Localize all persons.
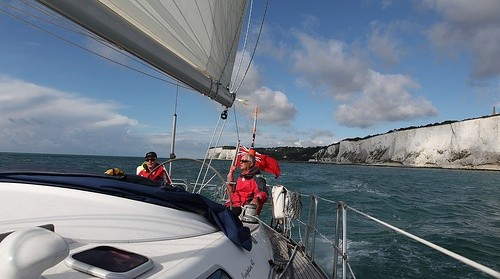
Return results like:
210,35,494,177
136,152,172,185
225,154,268,217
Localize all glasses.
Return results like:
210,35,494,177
240,160,251,163
146,158,155,162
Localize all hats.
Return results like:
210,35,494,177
144,151,157,160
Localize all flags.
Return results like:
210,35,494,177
234,144,280,179
